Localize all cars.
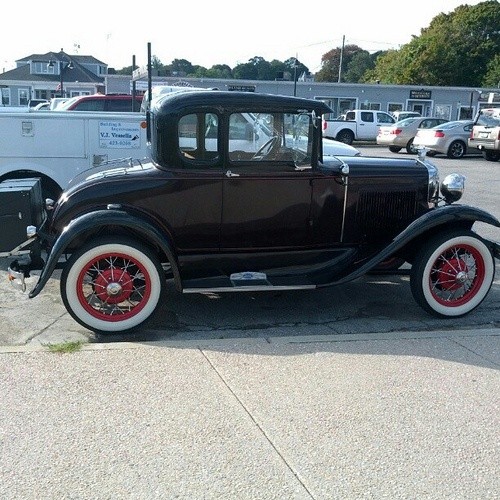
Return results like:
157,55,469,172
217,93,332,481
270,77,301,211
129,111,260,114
413,120,483,159
376,117,451,154
28,93,144,112
394,111,422,121
0,85,362,221
6,89,500,336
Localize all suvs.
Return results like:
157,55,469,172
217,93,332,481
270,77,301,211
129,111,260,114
468,108,500,160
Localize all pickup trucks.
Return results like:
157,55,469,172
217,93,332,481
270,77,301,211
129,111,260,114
322,110,398,145
